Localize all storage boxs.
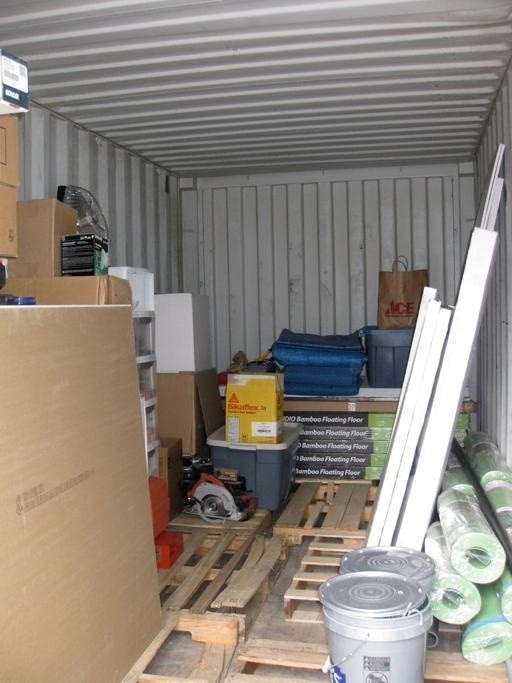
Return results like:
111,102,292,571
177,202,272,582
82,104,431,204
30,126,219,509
0,48,32,114
2,277,131,303
366,324,415,387
225,371,284,443
0,115,19,176
0,183,20,258
152,373,221,454
153,292,212,372
7,199,78,278
1,305,166,683
207,417,303,511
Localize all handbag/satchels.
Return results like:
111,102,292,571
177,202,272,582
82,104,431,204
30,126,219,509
378,255,428,329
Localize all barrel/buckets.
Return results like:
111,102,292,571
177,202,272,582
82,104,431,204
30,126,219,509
338,545,438,598
317,569,434,683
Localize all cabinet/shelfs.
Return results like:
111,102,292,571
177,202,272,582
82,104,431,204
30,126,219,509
130,310,160,478
